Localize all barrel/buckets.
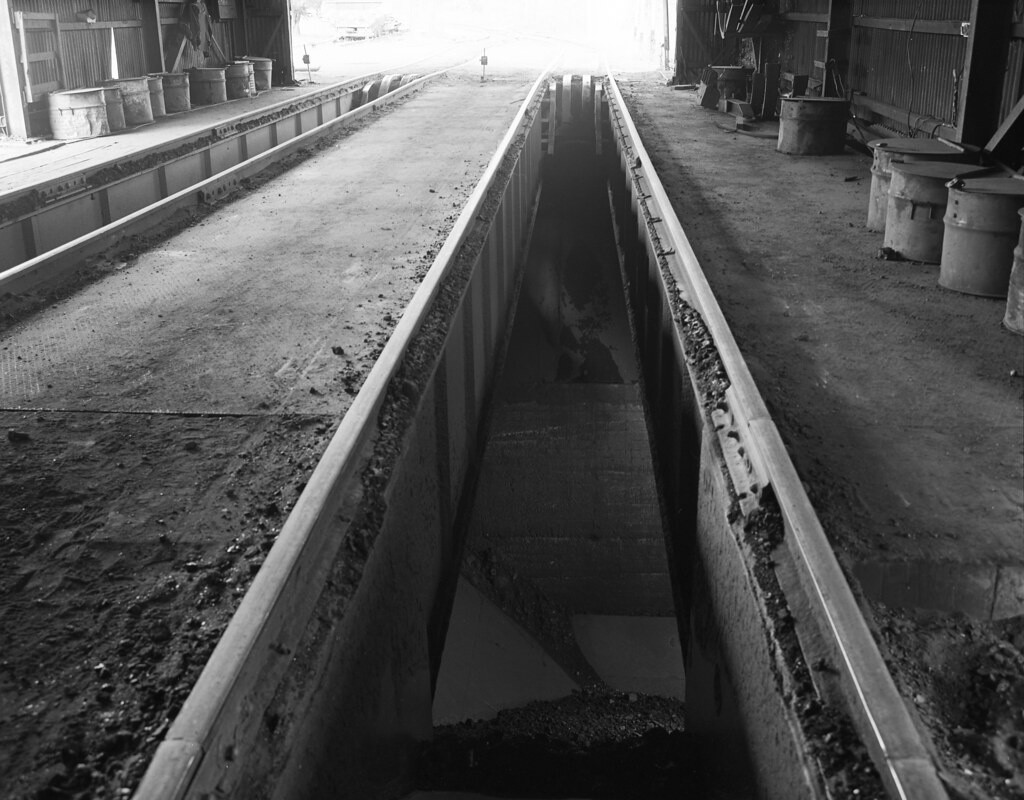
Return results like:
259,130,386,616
1002,208,1024,335
243,57,272,89
183,64,231,104
96,77,153,123
103,87,127,131
148,72,190,112
776,98,850,155
47,88,110,138
237,62,256,93
147,77,168,116
867,138,980,234
937,177,1024,296
227,65,251,98
881,159,986,264
